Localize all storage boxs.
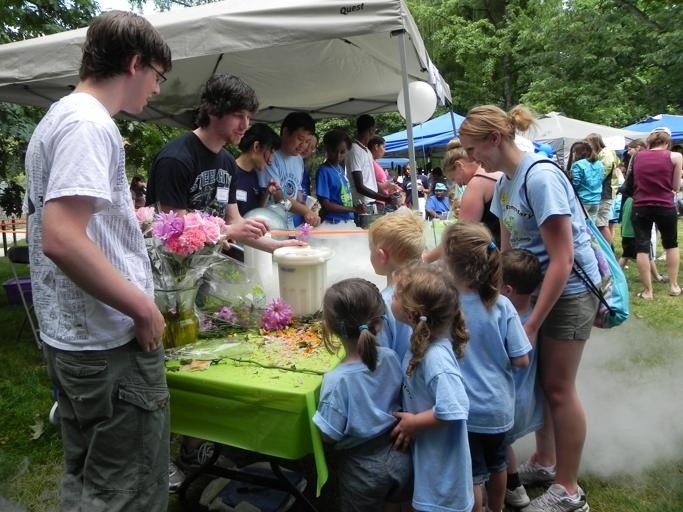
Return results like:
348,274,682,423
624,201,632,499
1,275,33,306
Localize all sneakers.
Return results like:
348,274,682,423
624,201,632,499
505,485,530,507
519,484,590,512
516,453,557,484
168,459,186,494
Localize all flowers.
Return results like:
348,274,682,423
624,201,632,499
297,222,315,244
194,290,294,340
130,202,236,348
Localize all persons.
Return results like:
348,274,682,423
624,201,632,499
129,75,321,254
313,104,629,511
24,10,172,512
567,127,682,301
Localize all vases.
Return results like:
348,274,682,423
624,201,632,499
150,279,202,353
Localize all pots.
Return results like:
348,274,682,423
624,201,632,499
359,214,382,228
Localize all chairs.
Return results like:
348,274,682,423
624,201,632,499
4,243,46,351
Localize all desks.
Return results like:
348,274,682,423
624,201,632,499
154,312,351,511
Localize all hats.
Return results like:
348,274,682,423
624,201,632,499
434,182,447,191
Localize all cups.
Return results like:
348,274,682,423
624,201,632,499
304,195,316,208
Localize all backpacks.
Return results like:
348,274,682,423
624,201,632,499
523,159,627,330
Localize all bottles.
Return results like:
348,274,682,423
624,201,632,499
391,191,400,210
417,196,426,220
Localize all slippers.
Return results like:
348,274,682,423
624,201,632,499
637,274,681,300
